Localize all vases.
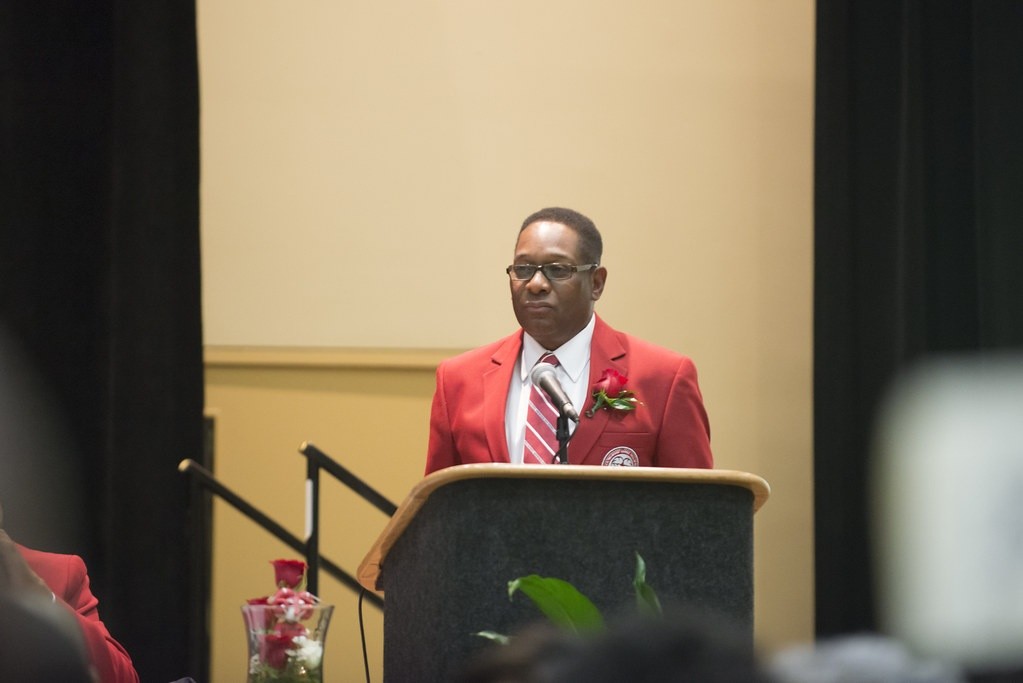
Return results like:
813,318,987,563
239,601,334,683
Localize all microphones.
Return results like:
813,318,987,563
531,363,580,423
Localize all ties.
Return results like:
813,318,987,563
523,354,561,463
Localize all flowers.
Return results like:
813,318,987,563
586,366,643,418
239,556,331,683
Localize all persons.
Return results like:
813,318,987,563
423,208,713,476
0,531,138,683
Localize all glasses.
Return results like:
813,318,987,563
506,262,598,281
